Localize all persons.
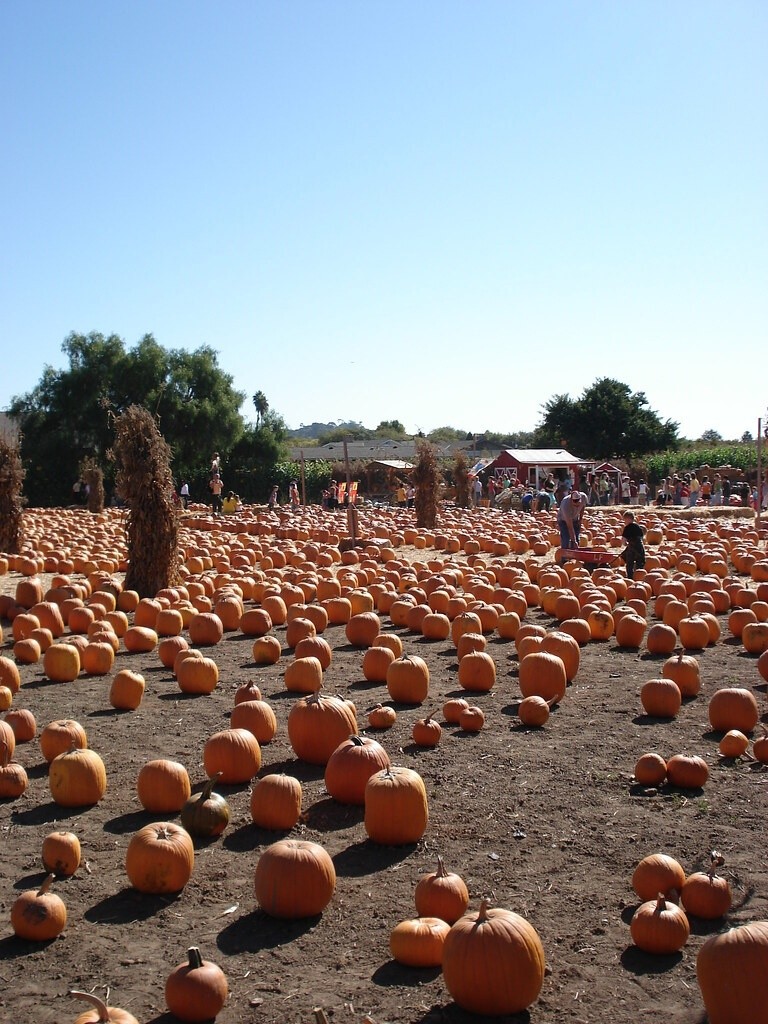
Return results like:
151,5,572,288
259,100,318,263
578,472,650,506
536,492,550,513
269,485,279,511
540,472,573,509
180,480,189,507
557,490,588,561
622,510,645,579
397,484,406,508
73,481,90,503
522,491,533,513
322,480,339,511
408,485,416,508
657,473,768,512
289,478,300,511
474,472,521,501
223,491,242,513
209,474,224,512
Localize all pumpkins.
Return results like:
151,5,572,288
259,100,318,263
697,920,768,1024
0,503,768,966
166,946,228,1018
69,989,138,1024
441,896,544,1012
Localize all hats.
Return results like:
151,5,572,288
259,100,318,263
571,491,581,499
